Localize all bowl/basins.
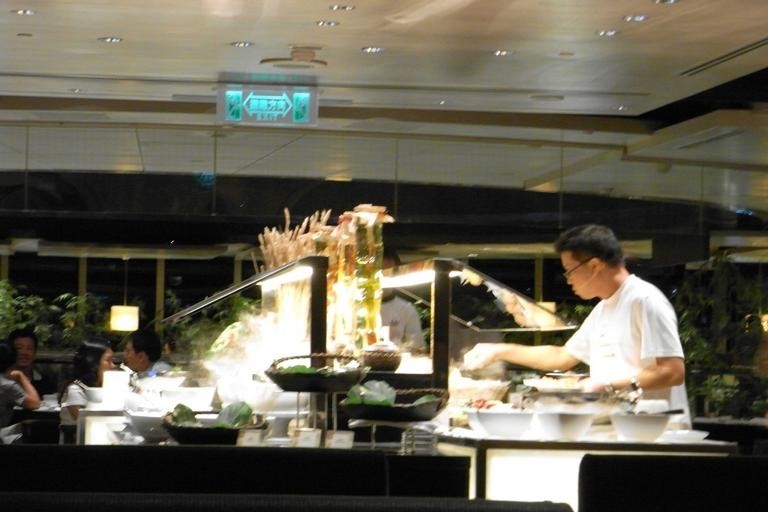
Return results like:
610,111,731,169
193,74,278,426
84,372,445,453
38,392,64,411
451,365,710,441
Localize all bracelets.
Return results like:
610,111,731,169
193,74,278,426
606,380,612,392
630,378,641,392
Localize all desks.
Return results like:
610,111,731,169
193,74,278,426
75,404,221,445
434,432,740,501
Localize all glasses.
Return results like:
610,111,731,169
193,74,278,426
562,255,599,281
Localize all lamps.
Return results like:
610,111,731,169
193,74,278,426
108,259,142,333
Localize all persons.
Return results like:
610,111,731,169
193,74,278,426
459,223,692,431
0,329,173,445
380,247,424,351
